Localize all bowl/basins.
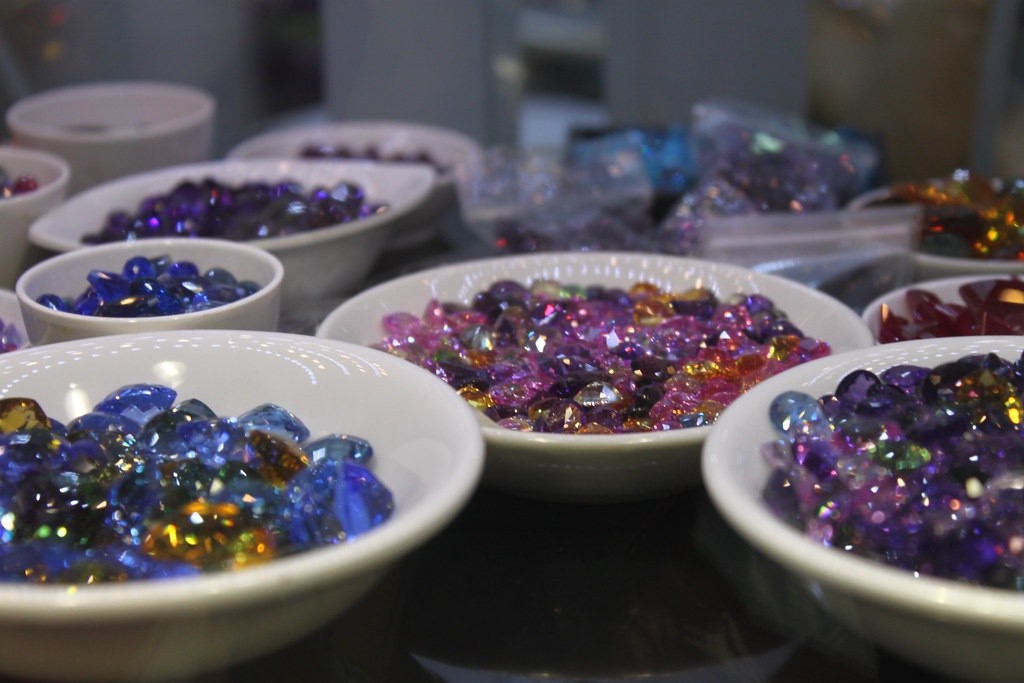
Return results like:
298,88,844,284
0,83,1024,683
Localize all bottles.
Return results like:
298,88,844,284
498,11,613,157
